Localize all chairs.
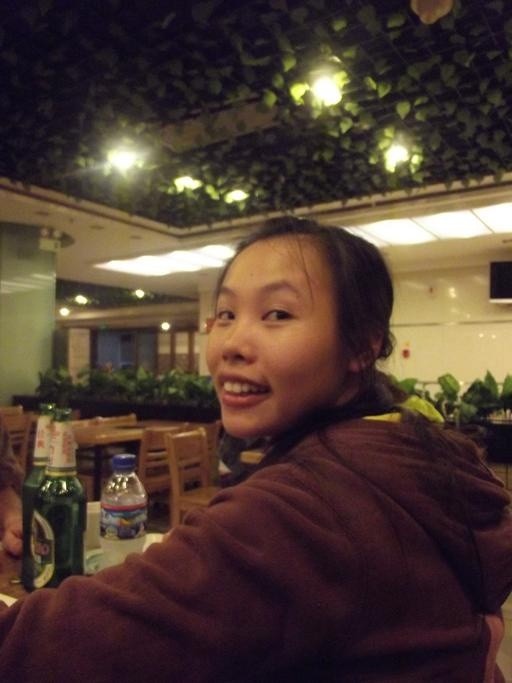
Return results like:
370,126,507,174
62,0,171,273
1,401,223,531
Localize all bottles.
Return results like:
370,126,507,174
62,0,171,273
20,403,150,594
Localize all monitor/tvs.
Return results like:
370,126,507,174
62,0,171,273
488,260,512,305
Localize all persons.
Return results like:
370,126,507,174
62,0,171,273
0,211,511,682
0,411,47,578
